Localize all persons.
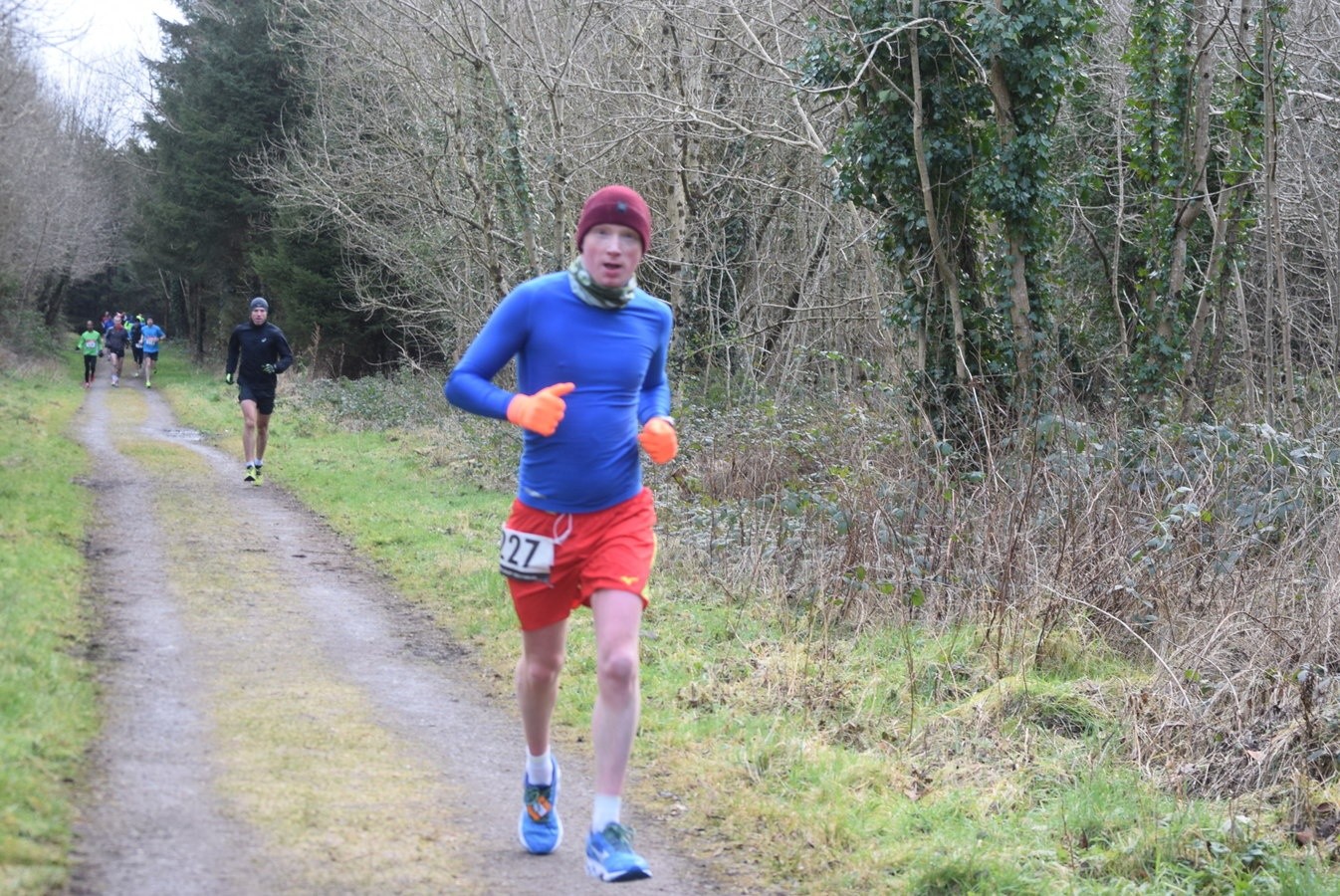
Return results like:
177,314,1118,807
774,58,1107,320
99,308,149,377
74,318,104,388
224,296,292,482
439,183,680,881
102,314,131,387
136,317,167,389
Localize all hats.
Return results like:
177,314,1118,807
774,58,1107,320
250,297,269,312
577,185,652,254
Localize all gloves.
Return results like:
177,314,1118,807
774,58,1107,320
262,363,276,375
639,417,678,466
75,347,79,351
98,350,103,357
226,373,234,384
505,383,575,437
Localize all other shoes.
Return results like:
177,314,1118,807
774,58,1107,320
111,381,119,387
135,372,140,377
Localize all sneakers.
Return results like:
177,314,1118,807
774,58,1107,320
90,375,94,382
244,465,256,482
146,382,151,388
518,753,563,855
585,822,652,882
254,466,264,486
85,382,90,389
153,369,158,374
